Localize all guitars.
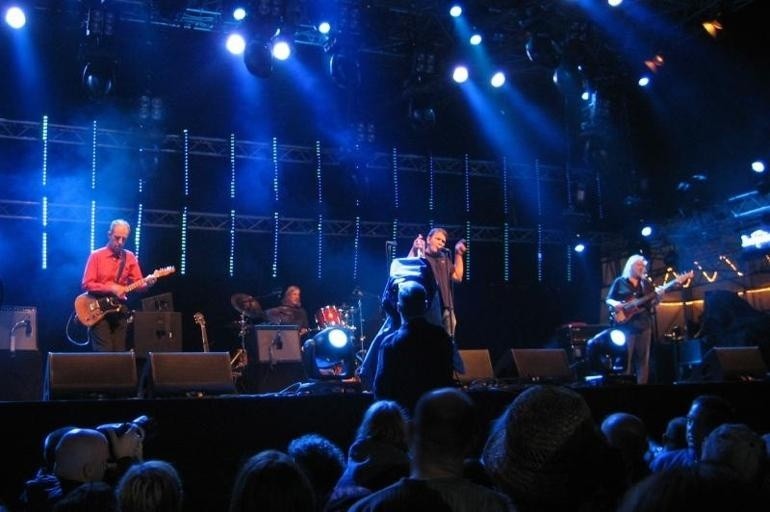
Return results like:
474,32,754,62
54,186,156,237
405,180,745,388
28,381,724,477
74,266,175,326
608,271,696,323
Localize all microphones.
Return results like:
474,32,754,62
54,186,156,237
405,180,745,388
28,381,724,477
437,246,449,251
644,272,656,287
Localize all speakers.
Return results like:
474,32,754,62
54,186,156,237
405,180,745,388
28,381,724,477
704,347,766,380
255,325,303,364
140,352,234,394
0,305,38,352
453,349,497,382
44,352,137,400
509,348,572,380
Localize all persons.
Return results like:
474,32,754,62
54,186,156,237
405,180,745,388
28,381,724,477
372,279,466,417
605,253,666,387
406,226,468,373
261,284,310,344
19,382,769,511
79,218,158,351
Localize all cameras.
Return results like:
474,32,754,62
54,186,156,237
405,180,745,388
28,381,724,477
96,414,151,442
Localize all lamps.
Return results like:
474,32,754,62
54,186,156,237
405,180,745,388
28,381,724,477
563,229,588,254
750,149,768,188
301,323,355,382
635,212,659,243
441,3,508,90
220,1,292,81
584,329,630,385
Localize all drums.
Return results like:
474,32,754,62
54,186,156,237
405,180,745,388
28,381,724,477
315,305,345,328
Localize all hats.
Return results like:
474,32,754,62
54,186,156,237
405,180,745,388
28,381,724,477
485,383,618,503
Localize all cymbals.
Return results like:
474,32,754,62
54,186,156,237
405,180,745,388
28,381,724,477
232,294,262,317
